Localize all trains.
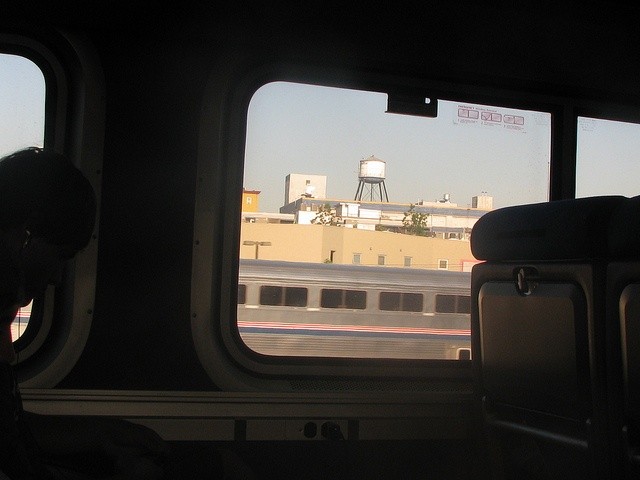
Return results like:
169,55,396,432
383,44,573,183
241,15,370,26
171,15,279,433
0,0,640,479
237,258,472,361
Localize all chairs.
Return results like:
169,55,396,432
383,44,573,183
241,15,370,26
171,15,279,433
618,195,639,478
470,195,632,479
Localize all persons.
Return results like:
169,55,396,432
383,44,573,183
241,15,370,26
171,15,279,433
1,145,172,480
